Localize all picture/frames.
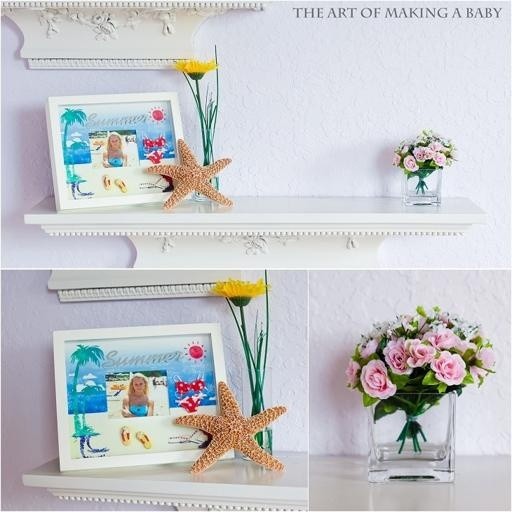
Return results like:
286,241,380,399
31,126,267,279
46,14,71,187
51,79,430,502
42,93,193,212
54,323,234,472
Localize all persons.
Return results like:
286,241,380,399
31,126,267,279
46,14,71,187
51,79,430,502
120,373,155,417
100,132,128,168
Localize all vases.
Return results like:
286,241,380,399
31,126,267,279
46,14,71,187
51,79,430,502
194,129,224,204
401,169,443,207
366,392,457,484
240,369,274,461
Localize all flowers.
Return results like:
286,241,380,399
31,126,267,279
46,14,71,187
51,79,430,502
172,43,226,193
206,270,273,456
392,128,459,196
342,306,498,458
